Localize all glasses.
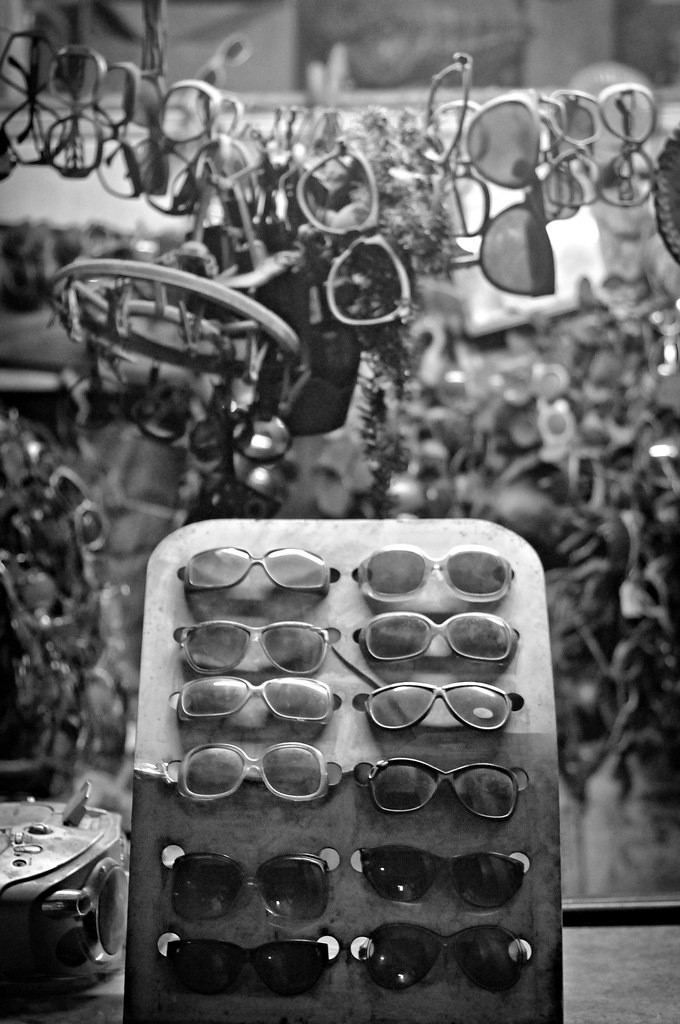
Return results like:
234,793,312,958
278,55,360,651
183,544,330,597
360,844,524,908
358,923,526,991
176,674,333,727
0,340,680,794
364,681,513,730
170,851,329,922
0,24,661,334
366,757,518,820
357,611,516,662
356,544,512,603
178,742,329,801
181,619,329,675
166,938,328,996
